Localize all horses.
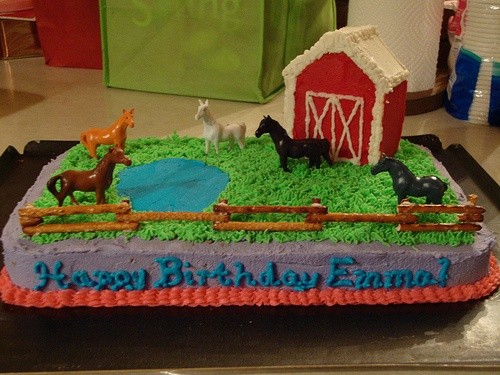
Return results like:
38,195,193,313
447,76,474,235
46,146,132,207
194,98,247,154
371,155,448,205
255,115,335,171
80,107,136,159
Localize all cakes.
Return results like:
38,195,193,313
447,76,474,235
0,27,496,292
